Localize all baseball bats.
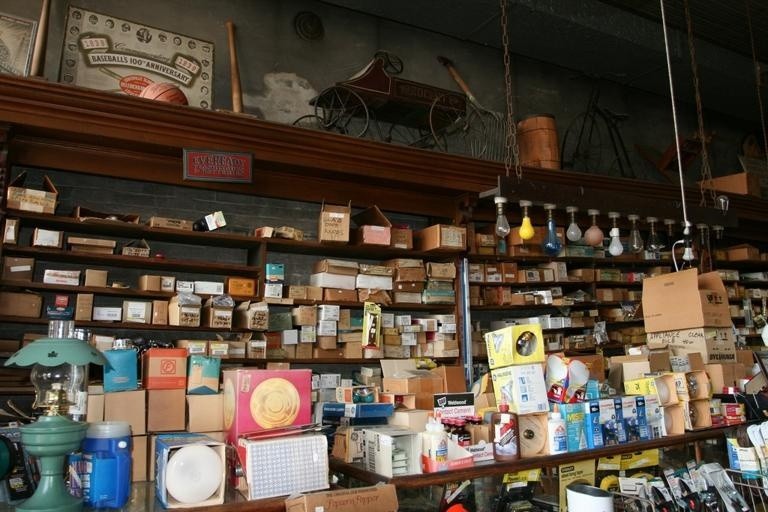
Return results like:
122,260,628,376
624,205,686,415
226,20,245,115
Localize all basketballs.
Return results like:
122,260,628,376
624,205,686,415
139,82,188,106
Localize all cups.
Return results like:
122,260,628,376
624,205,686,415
85,420,133,508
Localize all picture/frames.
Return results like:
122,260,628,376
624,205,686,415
57,3,215,112
0,12,38,79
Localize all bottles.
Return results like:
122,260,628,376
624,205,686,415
491,404,522,463
423,414,483,462
